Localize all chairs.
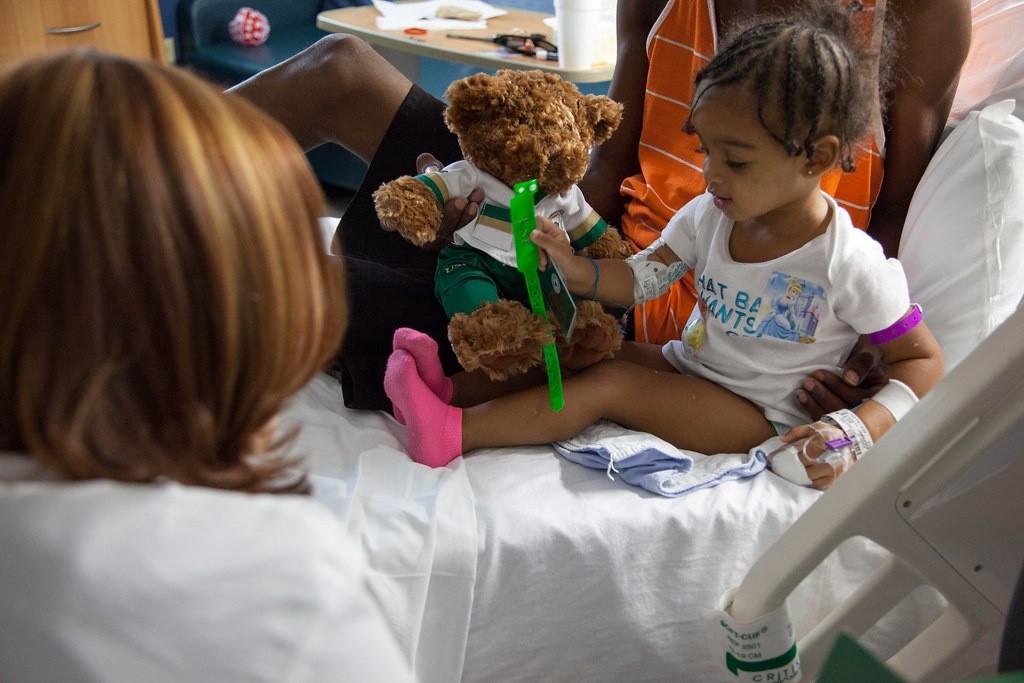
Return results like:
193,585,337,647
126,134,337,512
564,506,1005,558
179,0,336,87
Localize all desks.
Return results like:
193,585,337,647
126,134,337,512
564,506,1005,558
318,0,624,86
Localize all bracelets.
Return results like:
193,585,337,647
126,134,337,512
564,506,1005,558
823,408,873,460
574,256,599,299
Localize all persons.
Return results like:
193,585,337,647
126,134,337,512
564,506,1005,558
385,14,944,491
211,0,970,422
0,55,423,683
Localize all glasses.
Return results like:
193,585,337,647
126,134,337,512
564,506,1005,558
493,34,558,57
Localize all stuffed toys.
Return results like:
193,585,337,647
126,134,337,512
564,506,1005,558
371,71,633,382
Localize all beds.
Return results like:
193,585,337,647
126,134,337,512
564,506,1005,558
262,216,1024,683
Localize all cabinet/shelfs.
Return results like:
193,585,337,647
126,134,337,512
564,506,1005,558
0,0,168,76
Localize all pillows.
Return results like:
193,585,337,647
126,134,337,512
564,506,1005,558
886,96,1024,374
